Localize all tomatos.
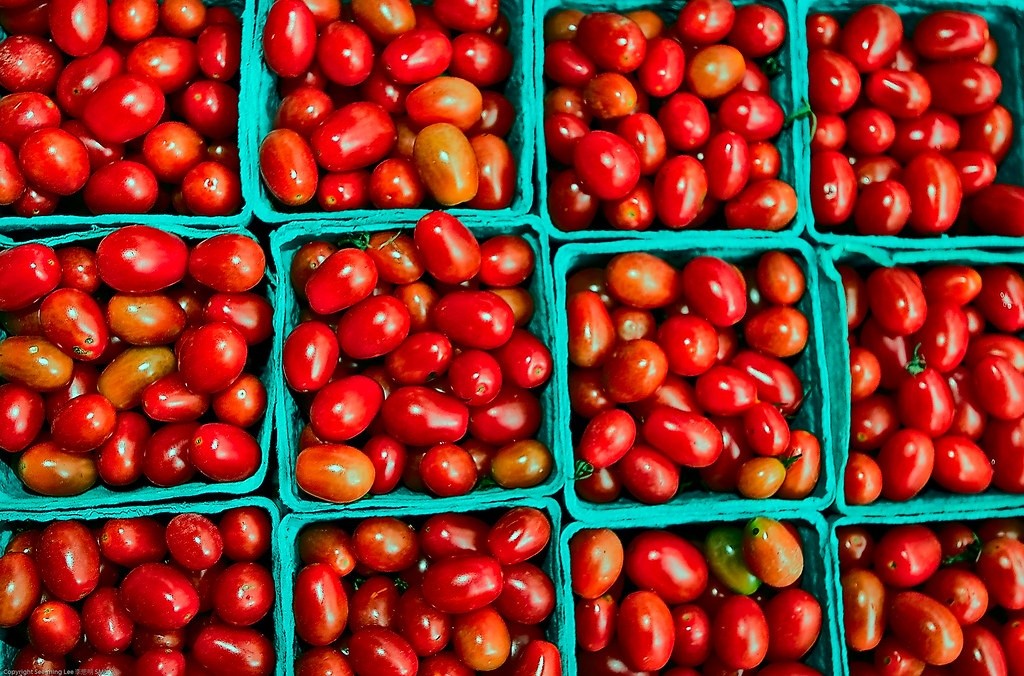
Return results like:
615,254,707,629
0,0,1024,676
